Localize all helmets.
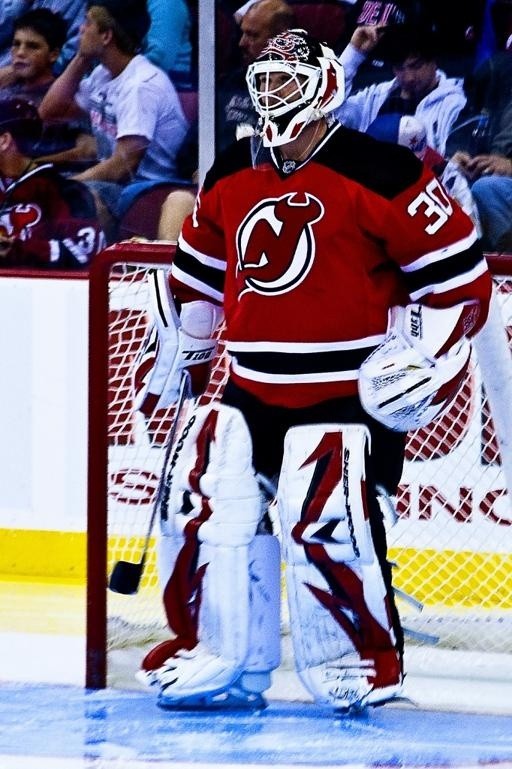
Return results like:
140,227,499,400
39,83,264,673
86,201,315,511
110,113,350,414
248,28,348,149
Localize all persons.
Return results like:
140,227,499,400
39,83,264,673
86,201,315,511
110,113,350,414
128,25,493,721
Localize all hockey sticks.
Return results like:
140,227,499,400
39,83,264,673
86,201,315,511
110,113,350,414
111,377,185,594
